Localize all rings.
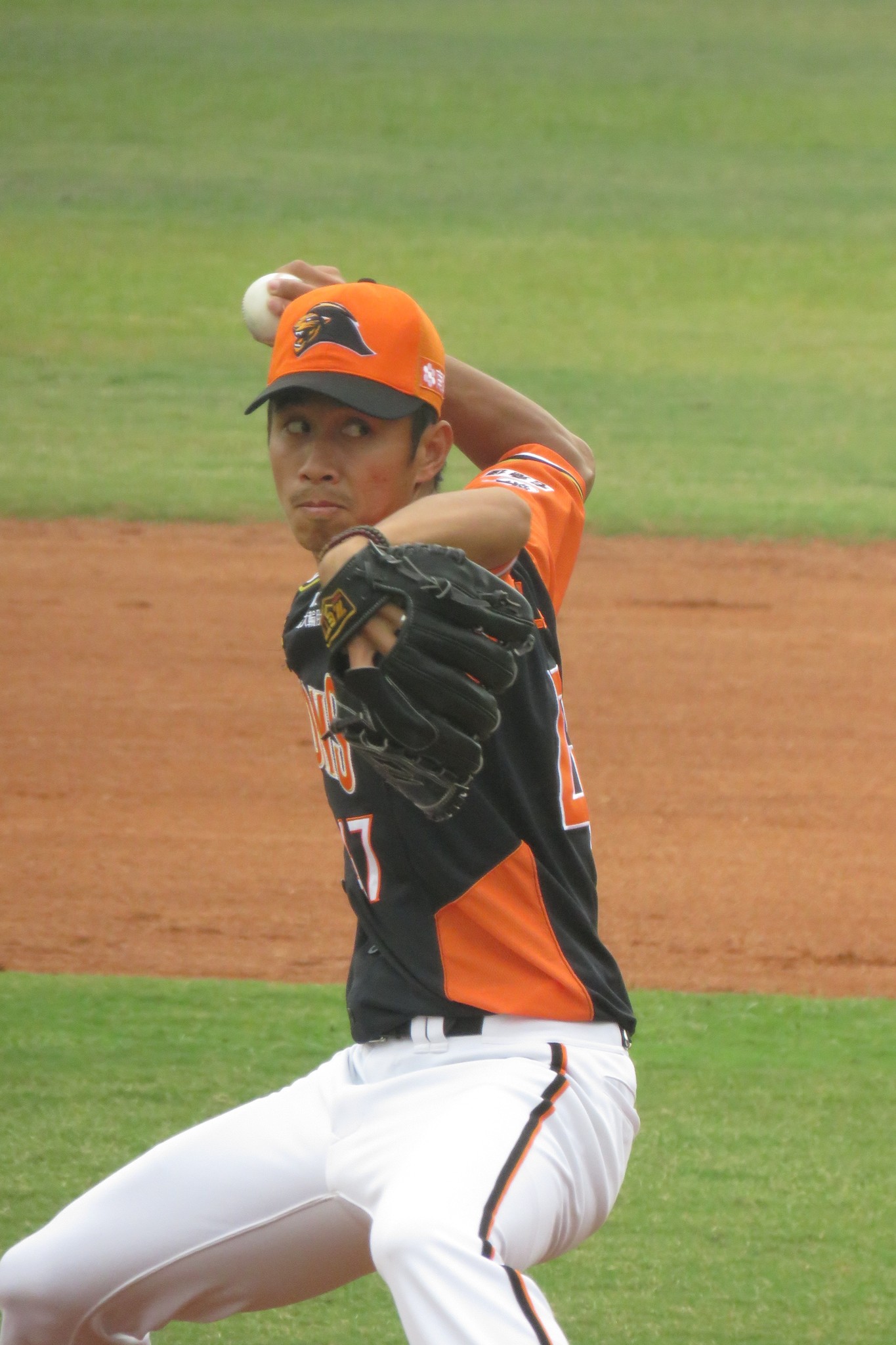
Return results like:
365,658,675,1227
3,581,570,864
397,612,407,632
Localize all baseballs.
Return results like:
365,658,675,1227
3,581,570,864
240,272,304,344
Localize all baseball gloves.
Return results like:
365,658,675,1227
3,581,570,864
315,539,534,823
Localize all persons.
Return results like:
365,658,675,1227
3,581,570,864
0,258,641,1345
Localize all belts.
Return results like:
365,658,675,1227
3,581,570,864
381,1013,631,1050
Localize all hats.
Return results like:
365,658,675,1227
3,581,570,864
243,277,447,420
